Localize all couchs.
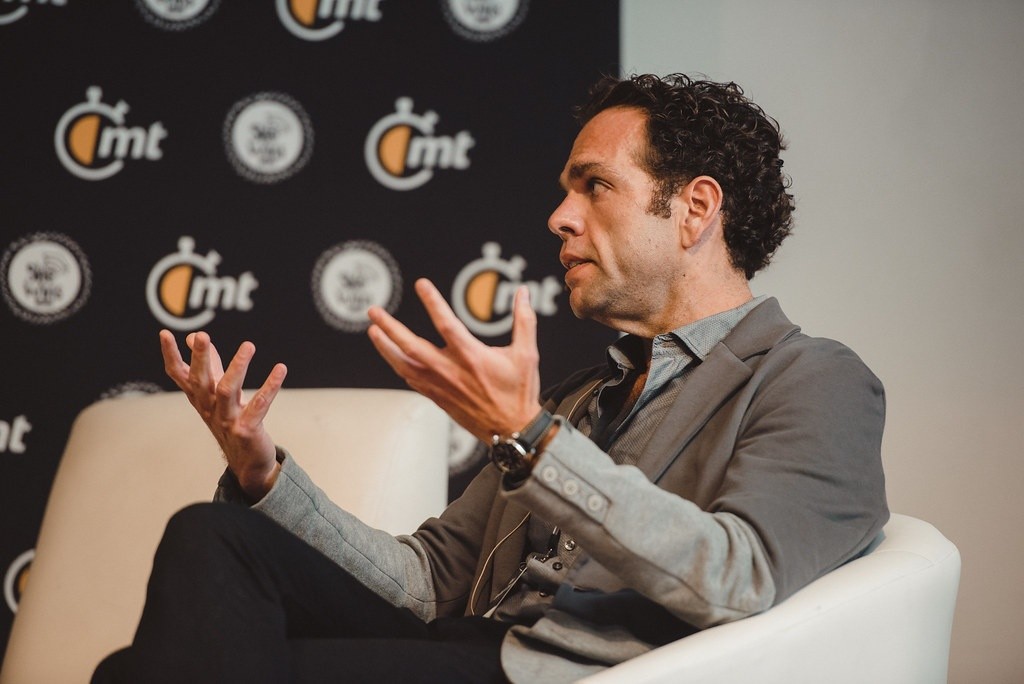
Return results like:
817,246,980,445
578,513,962,684
0,390,451,684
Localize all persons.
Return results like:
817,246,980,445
89,71,891,684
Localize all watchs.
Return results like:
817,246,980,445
487,408,554,473
